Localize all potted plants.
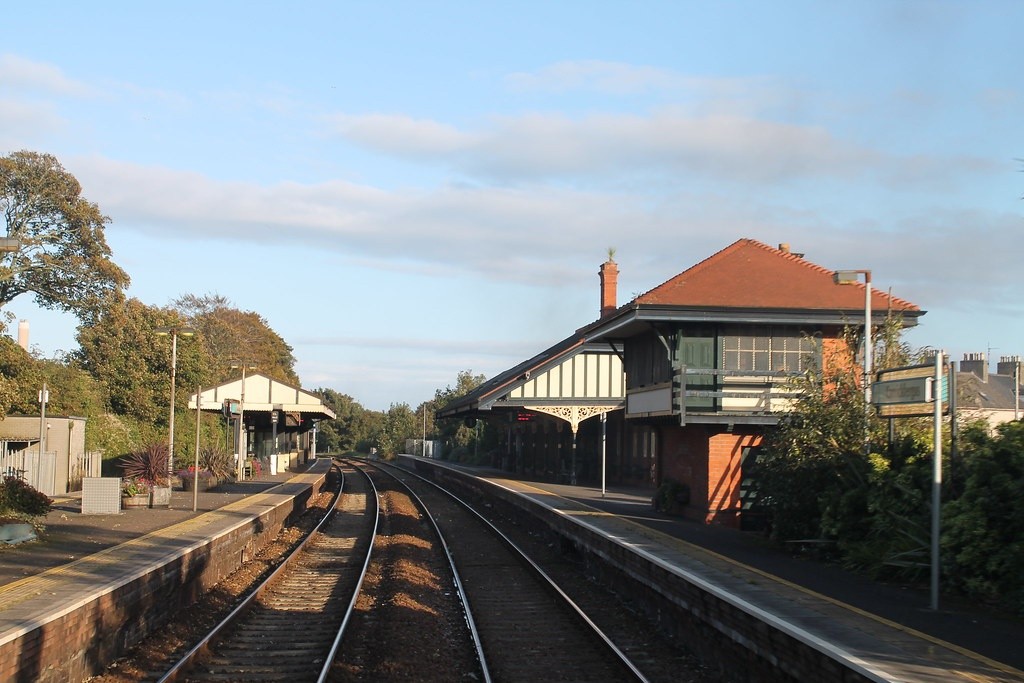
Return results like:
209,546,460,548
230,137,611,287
116,440,181,509
199,446,234,491
246,456,252,467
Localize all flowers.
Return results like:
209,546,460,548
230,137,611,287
176,466,201,480
189,470,212,479
123,478,156,496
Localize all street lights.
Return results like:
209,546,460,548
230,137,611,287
230,361,258,484
152,326,193,497
830,268,876,442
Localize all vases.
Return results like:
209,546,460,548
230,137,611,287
191,477,217,491
182,479,191,492
121,493,150,510
